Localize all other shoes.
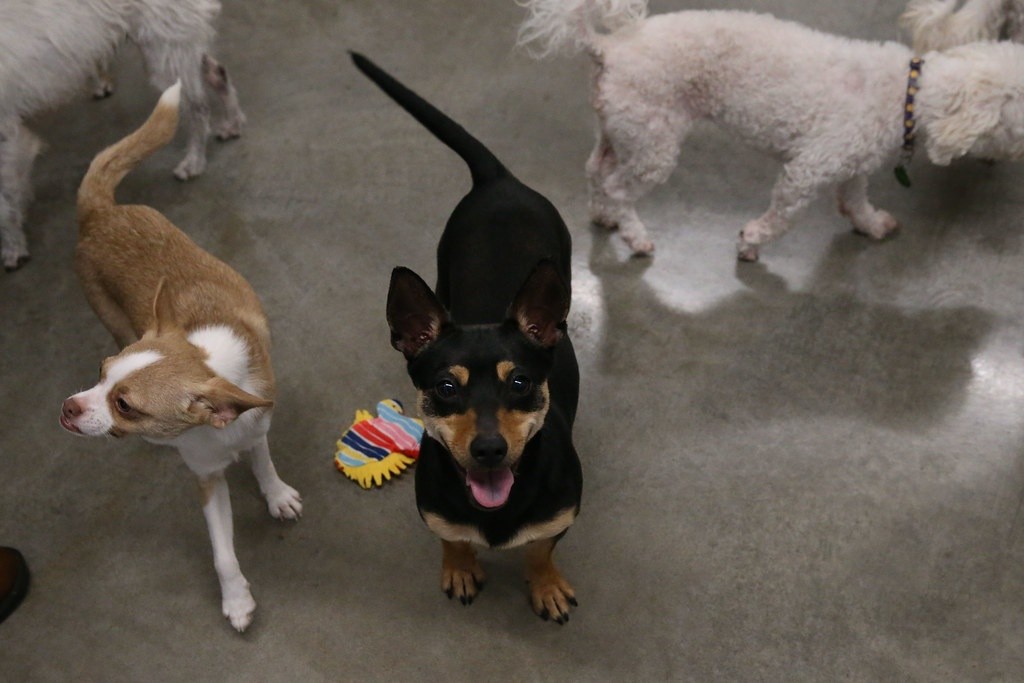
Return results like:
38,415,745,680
0,546,29,622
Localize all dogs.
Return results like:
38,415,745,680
339,47,586,628
509,1,1024,265
1,0,306,636
